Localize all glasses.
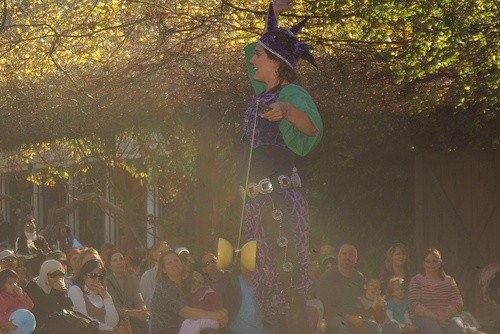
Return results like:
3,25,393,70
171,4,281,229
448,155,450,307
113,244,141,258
88,272,103,281
47,273,65,278
203,258,217,266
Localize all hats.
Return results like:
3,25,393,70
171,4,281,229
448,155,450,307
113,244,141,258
256,2,319,71
1,249,17,262
39,259,66,284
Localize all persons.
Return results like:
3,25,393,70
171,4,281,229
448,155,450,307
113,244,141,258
385,277,418,334
68,260,120,334
374,242,418,294
343,274,389,334
35,259,74,334
0,212,263,334
0,269,35,334
316,241,384,334
150,250,227,334
407,248,465,334
237,1,324,334
103,250,150,334
199,252,232,295
